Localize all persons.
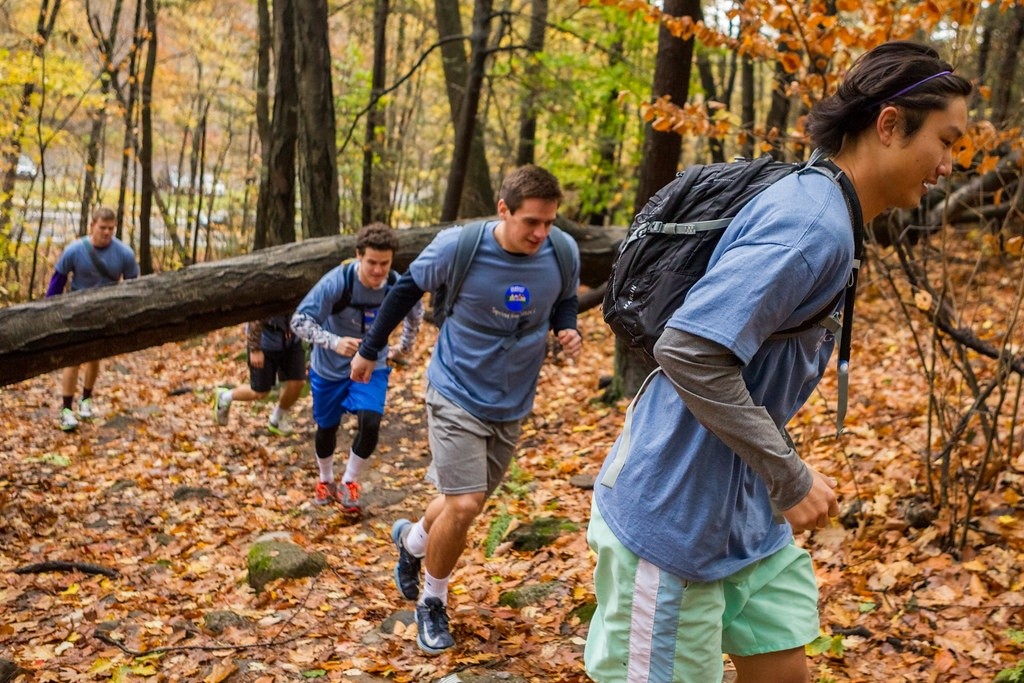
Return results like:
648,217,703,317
349,163,583,656
292,223,425,514
585,41,973,683
46,208,139,430
213,314,306,437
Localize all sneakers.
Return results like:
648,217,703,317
315,479,337,506
58,408,79,431
414,596,458,655
267,416,297,438
213,387,231,426
81,397,94,419
337,477,362,513
391,518,423,601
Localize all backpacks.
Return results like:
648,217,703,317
596,154,854,439
428,219,571,335
